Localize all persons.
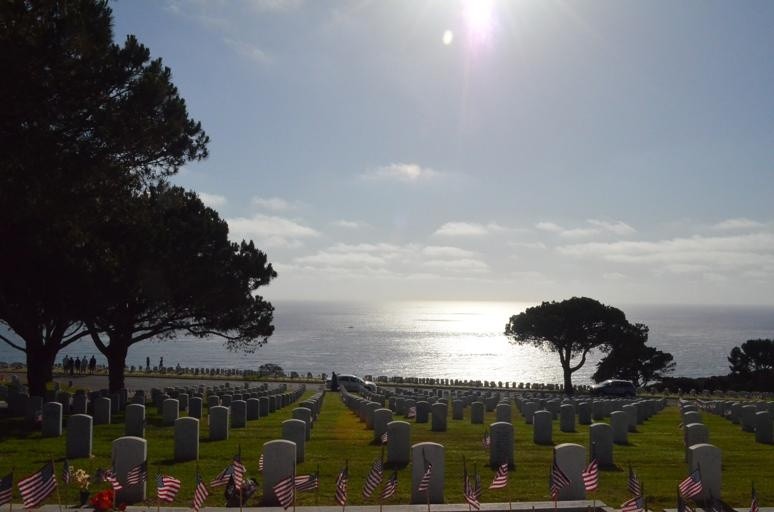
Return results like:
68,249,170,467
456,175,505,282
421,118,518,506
62,354,68,374
81,355,87,374
328,371,338,393
68,357,73,374
75,356,80,374
89,354,95,373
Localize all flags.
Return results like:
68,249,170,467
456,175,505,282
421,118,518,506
418,460,432,491
33,409,42,422
678,468,703,499
487,463,509,489
481,426,490,446
257,452,264,472
748,485,760,512
380,430,391,443
581,458,600,491
362,456,398,499
550,462,570,499
618,470,644,511
407,406,416,417
274,469,350,509
464,472,482,509
1,455,257,511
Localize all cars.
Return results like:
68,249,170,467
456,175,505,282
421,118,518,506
325,374,377,394
587,378,636,398
